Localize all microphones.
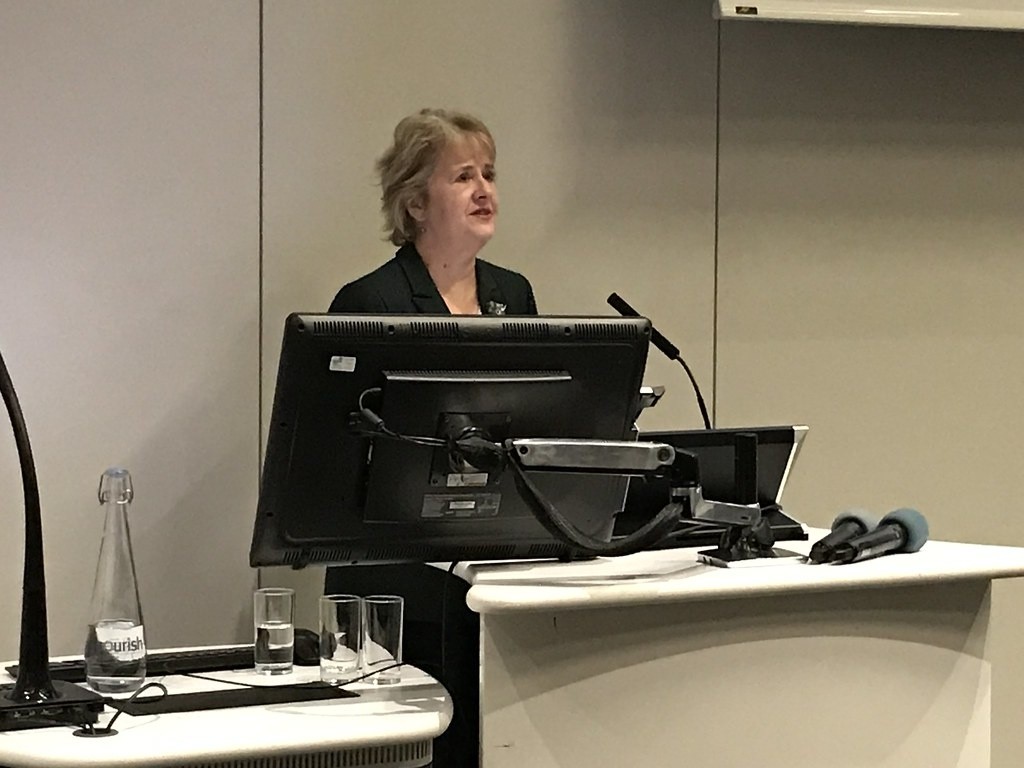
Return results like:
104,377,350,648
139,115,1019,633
810,510,875,562
607,292,711,431
834,507,929,563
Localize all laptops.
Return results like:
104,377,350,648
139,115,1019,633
610,425,811,547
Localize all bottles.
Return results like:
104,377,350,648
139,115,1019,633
85,470,147,694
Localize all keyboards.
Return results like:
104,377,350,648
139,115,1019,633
6,647,258,685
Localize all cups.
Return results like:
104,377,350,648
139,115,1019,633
318,595,361,687
361,596,405,685
253,587,296,675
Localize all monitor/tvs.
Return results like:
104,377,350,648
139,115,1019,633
250,312,653,570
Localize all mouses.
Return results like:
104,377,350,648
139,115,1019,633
293,628,320,666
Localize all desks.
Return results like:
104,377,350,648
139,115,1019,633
426,532,1024,767
0,642,453,768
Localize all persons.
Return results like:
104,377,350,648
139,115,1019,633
322,106,541,768
